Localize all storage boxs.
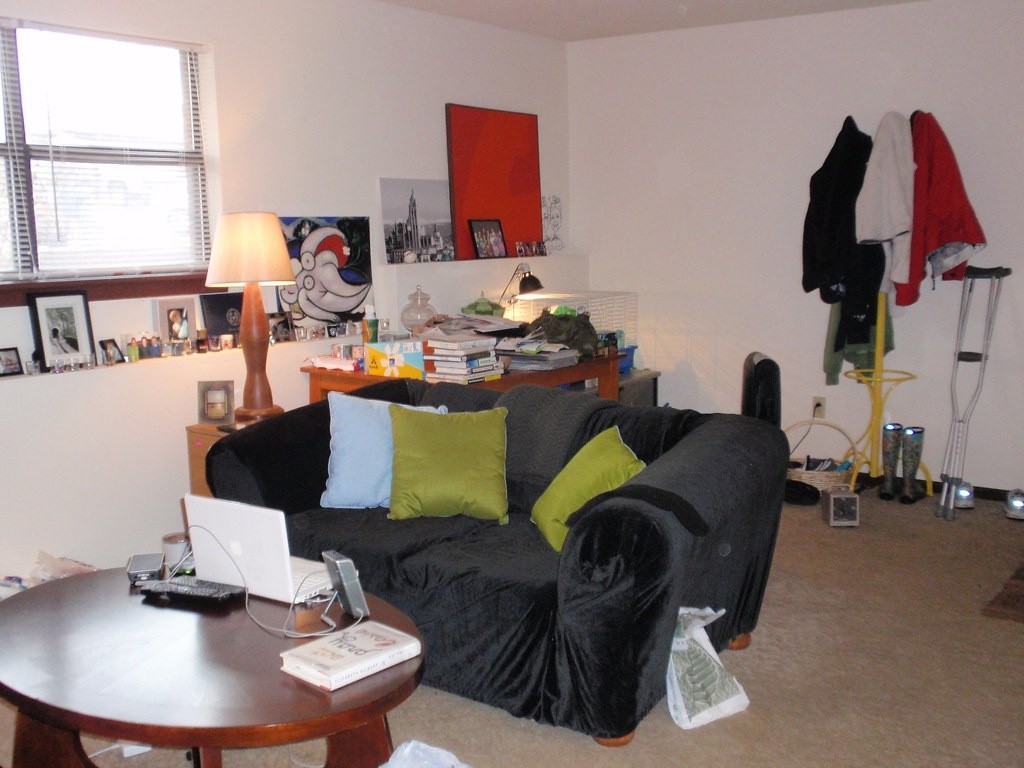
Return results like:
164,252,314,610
617,345,638,373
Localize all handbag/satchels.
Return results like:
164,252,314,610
665,607,749,729
529,305,598,356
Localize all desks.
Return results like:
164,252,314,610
0,567,425,768
618,370,661,406
301,351,625,403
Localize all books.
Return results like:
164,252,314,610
421,333,506,385
280,620,422,691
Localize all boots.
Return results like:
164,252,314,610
899,426,925,503
880,423,901,499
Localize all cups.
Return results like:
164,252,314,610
25,360,40,375
48,359,65,374
294,328,307,341
101,349,115,365
220,334,233,351
64,355,81,373
81,353,95,370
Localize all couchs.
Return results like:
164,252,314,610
207,378,788,745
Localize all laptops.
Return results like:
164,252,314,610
184,494,359,604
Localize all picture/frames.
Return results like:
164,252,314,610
469,219,509,259
99,337,125,364
26,289,99,372
0,347,24,376
157,298,197,344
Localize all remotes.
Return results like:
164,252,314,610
139,574,247,605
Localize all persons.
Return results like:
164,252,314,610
127,336,162,361
167,310,188,341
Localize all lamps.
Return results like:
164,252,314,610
498,262,544,304
205,212,297,430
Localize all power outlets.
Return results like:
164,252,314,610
813,396,825,419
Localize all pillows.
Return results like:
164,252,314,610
320,391,447,509
386,403,511,526
531,425,648,552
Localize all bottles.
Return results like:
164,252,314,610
362,304,379,344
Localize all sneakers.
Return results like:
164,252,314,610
954,482,975,508
1004,489,1024,519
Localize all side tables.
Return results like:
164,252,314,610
186,422,235,497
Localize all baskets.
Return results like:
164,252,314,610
783,421,857,493
